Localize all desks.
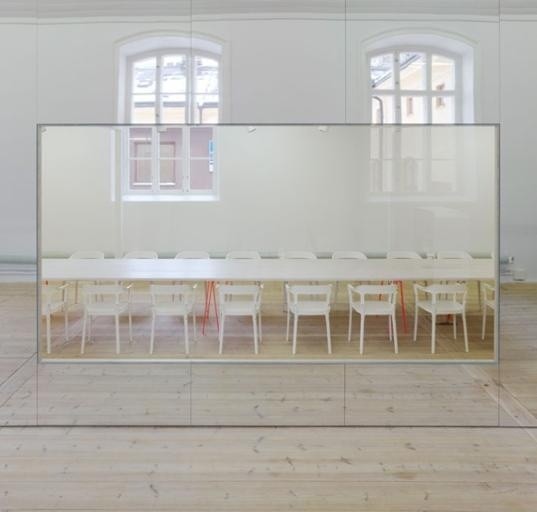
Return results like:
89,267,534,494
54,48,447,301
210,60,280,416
39,260,493,339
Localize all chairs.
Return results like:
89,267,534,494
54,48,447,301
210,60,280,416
481,283,494,341
346,282,400,354
170,249,212,306
215,283,264,354
38,283,71,354
386,250,429,303
412,282,471,354
149,282,199,354
330,250,371,304
223,250,264,304
64,249,105,306
277,249,320,302
434,249,478,302
79,283,135,355
118,249,160,304
284,283,333,355
